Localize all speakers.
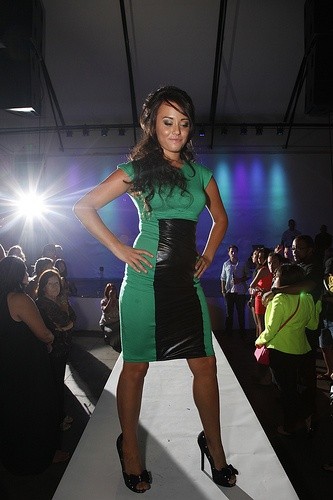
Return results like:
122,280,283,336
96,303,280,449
0,0,45,119
304,0,333,116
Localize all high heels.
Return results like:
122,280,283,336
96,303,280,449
197,430,239,488
116,433,152,493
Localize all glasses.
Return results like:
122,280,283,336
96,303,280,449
290,247,306,252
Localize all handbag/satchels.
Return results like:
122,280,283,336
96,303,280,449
254,344,270,367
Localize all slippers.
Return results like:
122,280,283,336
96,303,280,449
317,373,331,380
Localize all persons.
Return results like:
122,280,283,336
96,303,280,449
99,283,120,346
246,218,333,469
221,244,253,339
72,85,240,493
0,241,77,466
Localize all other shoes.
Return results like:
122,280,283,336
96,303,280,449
304,423,319,440
281,426,296,440
